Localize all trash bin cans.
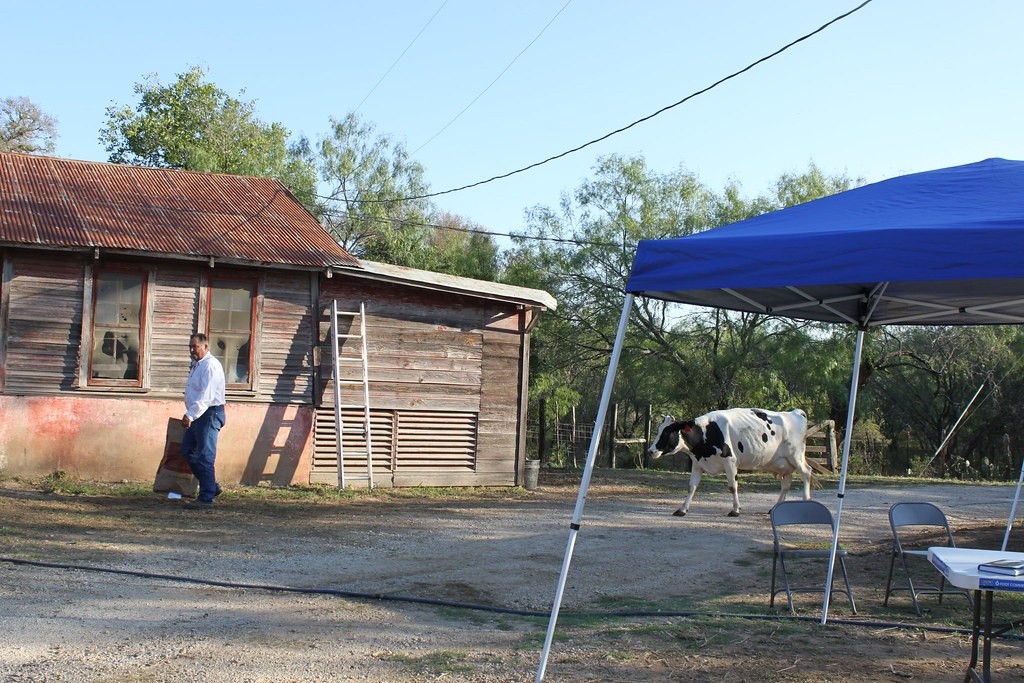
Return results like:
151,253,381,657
523,458,541,490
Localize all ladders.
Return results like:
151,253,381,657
327,297,375,490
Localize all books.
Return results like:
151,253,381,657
978,556,1024,578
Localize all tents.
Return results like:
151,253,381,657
534,155,1024,682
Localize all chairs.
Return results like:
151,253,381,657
884,500,974,618
770,500,857,617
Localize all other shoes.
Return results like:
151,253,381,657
184,500,214,509
214,483,223,496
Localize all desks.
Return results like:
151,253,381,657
925,546,1024,683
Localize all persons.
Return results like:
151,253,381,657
181,333,226,509
